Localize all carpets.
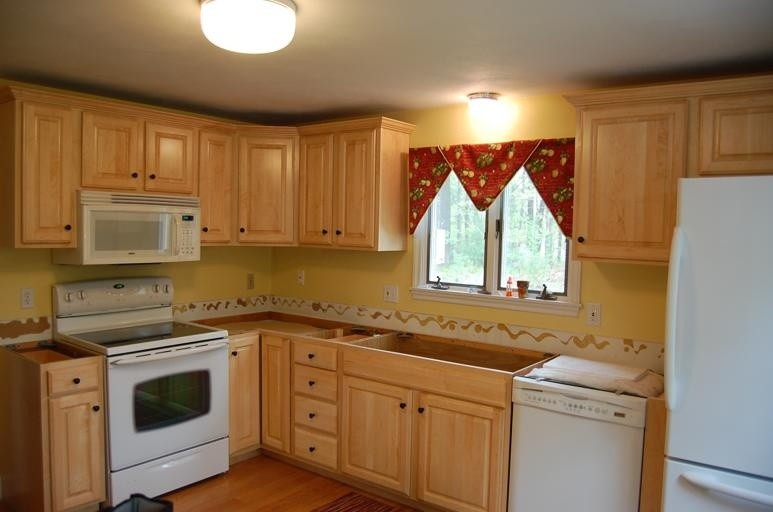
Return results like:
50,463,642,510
311,490,410,512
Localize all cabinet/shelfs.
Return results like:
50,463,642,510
695,74,773,176
75,92,198,197
561,81,694,267
1,83,79,250
298,116,416,254
235,121,297,246
293,320,394,483
343,332,561,512
194,114,235,247
1,338,109,512
191,316,327,468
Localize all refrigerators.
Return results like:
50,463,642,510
661,174,773,512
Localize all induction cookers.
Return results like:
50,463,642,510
56,305,229,359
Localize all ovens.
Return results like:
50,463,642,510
101,338,231,510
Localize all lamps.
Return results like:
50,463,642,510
468,90,506,136
198,0,299,56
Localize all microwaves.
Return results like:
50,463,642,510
50,189,203,267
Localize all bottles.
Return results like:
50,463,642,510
505,276,513,297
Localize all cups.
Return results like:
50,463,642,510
517,280,530,298
